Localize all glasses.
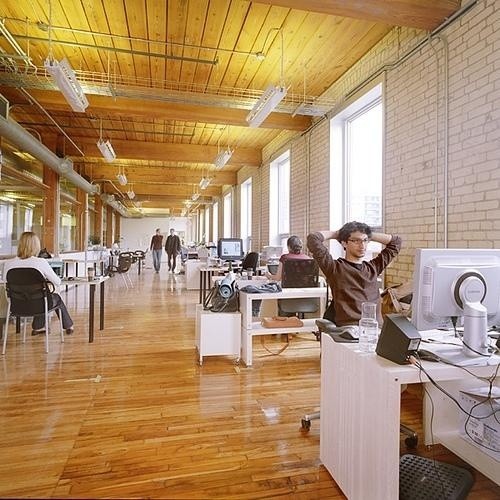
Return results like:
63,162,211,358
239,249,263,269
347,237,369,244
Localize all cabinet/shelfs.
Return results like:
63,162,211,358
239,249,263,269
195,303,241,367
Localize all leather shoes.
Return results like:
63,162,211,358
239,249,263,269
31,327,50,335
67,326,74,334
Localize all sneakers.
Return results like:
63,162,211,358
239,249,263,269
172,269,174,273
168,268,171,271
156,271,159,273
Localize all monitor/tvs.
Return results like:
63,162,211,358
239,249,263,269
410,247,500,365
267,263,279,273
217,238,243,262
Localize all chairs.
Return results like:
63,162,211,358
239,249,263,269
301,294,418,449
179,249,186,267
108,252,131,289
275,258,320,341
129,251,137,268
239,252,258,274
2,268,64,355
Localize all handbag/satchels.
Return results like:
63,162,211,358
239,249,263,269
203,281,240,313
261,317,303,328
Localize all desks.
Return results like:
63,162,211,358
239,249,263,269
0,275,109,343
62,257,106,292
237,285,389,367
319,325,500,499
183,256,241,290
212,273,326,306
198,262,286,305
110,254,143,275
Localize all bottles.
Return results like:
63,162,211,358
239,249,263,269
357,301,379,355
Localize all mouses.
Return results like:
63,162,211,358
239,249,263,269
67,276,74,282
340,328,358,339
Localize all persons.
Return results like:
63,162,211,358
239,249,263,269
306,221,402,330
165,229,181,274
3,232,76,337
150,228,163,273
265,236,312,282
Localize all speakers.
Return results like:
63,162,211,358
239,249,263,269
375,314,424,365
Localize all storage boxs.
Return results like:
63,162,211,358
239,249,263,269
459,384,500,465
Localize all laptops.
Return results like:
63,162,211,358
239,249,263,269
45,261,63,281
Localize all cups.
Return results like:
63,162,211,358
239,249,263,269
88,267,96,283
246,267,254,280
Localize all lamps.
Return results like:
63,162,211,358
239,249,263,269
42,3,89,113
191,185,201,202
96,118,116,163
246,29,288,131
114,164,128,185
125,182,136,200
213,126,233,171
200,167,213,190
